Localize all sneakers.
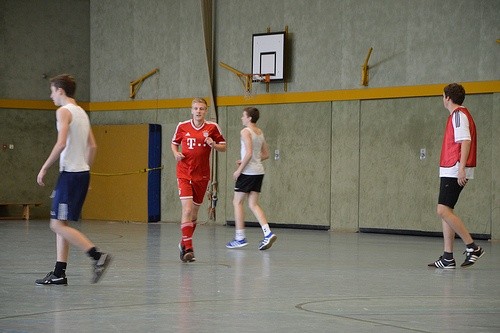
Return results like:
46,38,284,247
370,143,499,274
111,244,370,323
35,272,67,286
178,241,197,263
460,246,485,268
428,255,456,270
93,253,111,283
258,232,277,251
225,238,247,249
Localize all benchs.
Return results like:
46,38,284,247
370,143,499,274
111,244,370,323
0,202,41,219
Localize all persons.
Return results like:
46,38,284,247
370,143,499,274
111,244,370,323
34,74,110,286
427,83,486,269
171,97,226,262
225,107,277,251
